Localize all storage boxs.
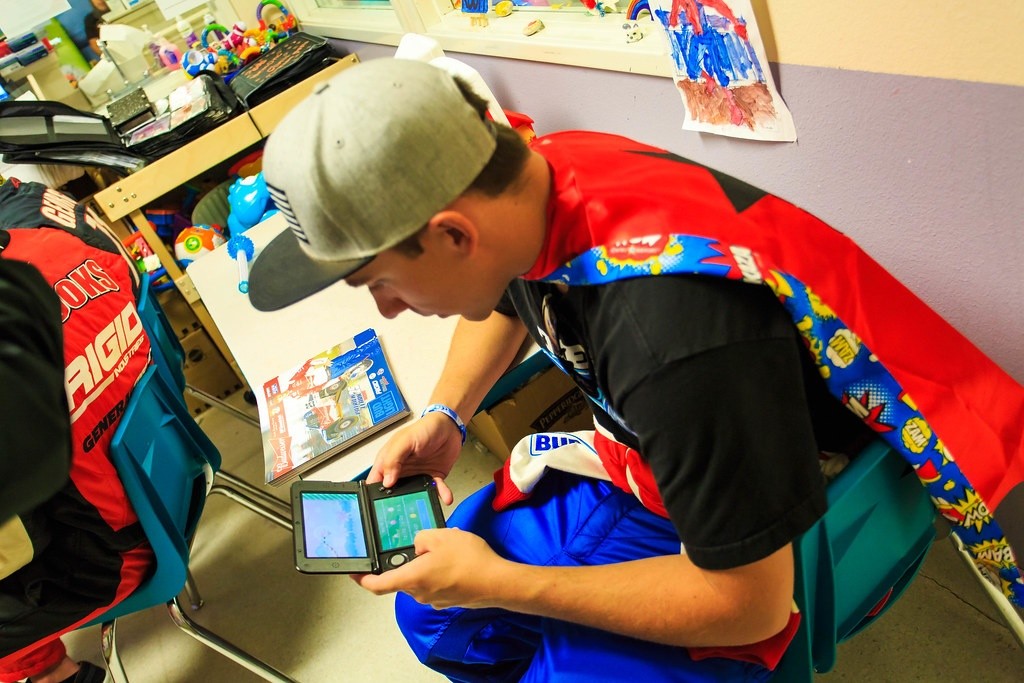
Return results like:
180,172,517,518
154,286,202,339
180,329,242,419
465,367,595,465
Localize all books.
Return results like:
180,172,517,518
255,328,410,490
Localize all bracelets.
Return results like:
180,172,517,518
420,403,467,445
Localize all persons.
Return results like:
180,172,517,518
261,57,901,683
0,174,164,683
84,0,111,56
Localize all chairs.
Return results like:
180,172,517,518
72,365,296,683
138,271,263,611
784,437,939,683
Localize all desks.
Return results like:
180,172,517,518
185,210,558,486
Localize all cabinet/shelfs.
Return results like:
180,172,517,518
92,53,361,395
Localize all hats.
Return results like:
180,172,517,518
247,56,497,312
